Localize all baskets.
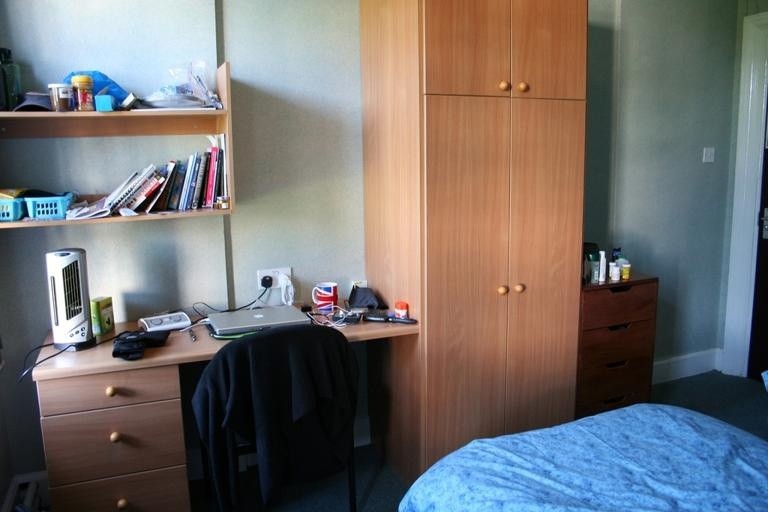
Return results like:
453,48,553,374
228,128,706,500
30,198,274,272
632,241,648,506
0,192,72,220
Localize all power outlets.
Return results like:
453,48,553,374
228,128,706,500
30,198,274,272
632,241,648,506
257,268,292,288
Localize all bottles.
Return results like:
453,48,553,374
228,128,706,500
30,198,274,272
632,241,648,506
48,75,95,112
395,302,407,319
217,196,230,210
0,47,22,111
580,244,631,284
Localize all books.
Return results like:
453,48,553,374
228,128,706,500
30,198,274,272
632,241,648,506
69,135,226,219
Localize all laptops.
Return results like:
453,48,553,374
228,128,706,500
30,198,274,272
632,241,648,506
207,304,312,336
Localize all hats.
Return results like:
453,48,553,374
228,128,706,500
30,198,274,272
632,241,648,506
13,93,53,112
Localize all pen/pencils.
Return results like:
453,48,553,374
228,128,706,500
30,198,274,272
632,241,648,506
189,328,196,341
389,318,417,324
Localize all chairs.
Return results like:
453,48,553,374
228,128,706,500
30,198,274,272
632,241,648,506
190,324,375,510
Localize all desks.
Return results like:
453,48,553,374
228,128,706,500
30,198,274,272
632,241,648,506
31,315,418,512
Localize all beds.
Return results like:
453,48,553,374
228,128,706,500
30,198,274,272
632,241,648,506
395,402,768,512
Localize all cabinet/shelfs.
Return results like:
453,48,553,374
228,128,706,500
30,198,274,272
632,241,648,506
359,2,588,491
575,269,660,419
0,62,236,229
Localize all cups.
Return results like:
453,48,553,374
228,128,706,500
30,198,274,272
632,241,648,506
310,282,339,318
